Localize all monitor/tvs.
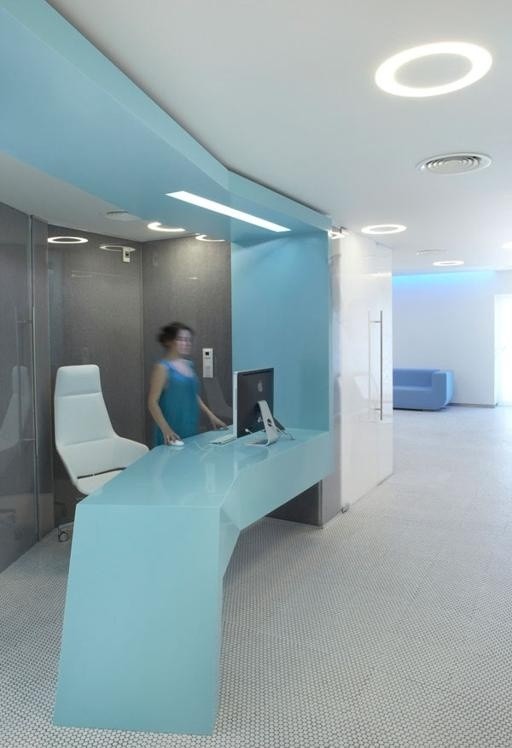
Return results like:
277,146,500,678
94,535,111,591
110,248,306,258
233,366,280,448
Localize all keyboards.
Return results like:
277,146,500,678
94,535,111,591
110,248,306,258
208,434,236,446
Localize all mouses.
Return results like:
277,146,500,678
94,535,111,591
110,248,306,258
169,436,185,447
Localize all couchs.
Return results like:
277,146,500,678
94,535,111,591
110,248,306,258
392,368,453,410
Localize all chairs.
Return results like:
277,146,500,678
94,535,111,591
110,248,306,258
0,365,32,452
53,364,151,541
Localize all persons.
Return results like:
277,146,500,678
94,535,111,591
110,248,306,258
146,320,230,447
